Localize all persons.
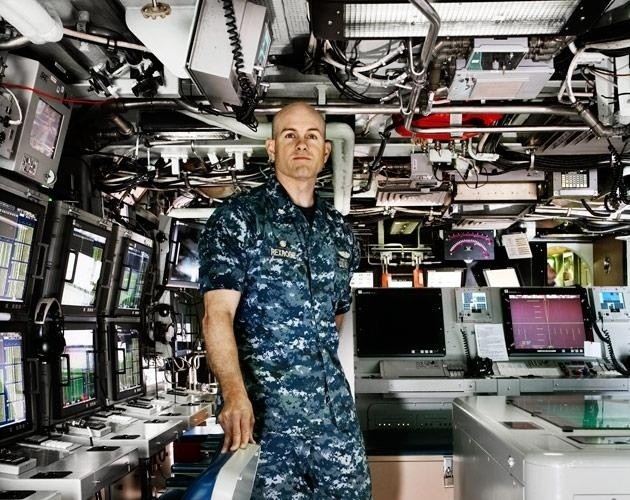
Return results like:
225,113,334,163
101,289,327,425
199,101,371,500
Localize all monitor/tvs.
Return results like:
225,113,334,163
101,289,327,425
105,316,146,404
354,289,448,357
57,321,102,421
105,224,154,318
0,328,36,445
47,198,112,322
0,178,49,328
165,219,208,289
28,97,64,159
502,284,592,359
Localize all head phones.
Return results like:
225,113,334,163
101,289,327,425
141,306,180,347
30,295,72,363
